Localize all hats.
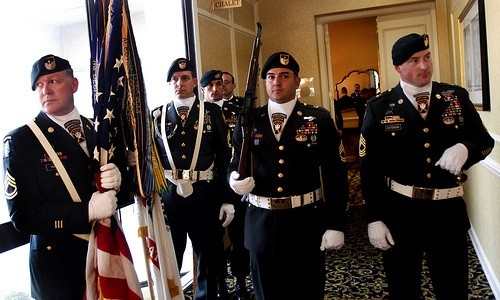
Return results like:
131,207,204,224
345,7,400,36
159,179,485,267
261,53,300,80
167,59,195,83
31,55,71,91
392,34,429,66
200,69,223,88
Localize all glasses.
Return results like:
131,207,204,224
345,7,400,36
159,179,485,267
223,80,233,85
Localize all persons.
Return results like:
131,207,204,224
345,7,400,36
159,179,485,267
2,55,123,300
227,51,349,300
334,83,375,135
149,58,248,300
357,33,495,300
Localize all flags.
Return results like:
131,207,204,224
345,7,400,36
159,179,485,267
84,0,185,300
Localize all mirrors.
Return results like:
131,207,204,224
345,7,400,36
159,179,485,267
335,68,381,100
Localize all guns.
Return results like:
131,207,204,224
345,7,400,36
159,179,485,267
238,22,263,180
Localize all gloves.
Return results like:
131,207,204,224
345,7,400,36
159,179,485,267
367,221,394,250
320,230,344,252
100,163,121,190
219,203,234,227
229,171,255,195
435,143,469,175
88,191,118,222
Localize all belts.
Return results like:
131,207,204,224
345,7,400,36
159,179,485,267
383,175,464,201
163,170,212,181
245,187,323,211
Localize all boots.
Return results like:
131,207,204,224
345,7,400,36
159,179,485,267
235,275,248,300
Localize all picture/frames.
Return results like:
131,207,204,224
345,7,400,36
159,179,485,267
457,0,493,112
